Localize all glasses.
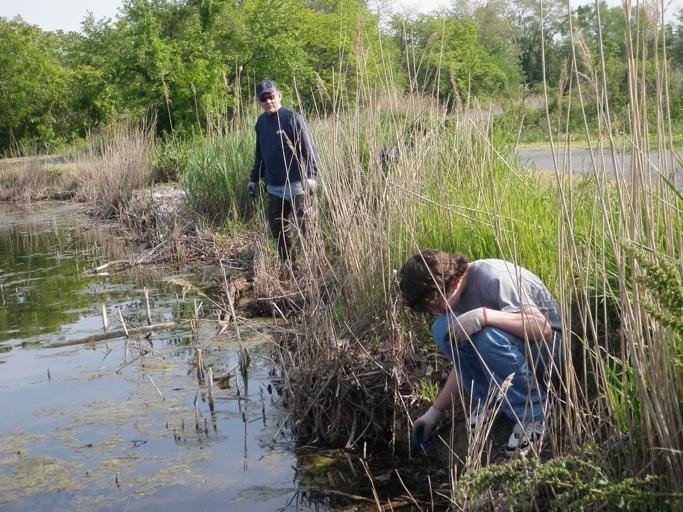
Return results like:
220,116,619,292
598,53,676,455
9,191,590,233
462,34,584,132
258,94,276,103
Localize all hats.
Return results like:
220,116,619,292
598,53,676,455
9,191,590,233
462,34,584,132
255,80,276,98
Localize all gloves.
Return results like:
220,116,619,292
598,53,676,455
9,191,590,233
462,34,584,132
305,178,316,195
246,181,257,198
412,406,442,444
446,307,488,344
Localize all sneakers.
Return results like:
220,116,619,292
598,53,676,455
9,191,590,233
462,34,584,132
465,405,502,434
505,419,548,458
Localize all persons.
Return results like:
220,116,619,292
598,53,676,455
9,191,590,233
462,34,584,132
245,80,317,276
399,249,575,458
